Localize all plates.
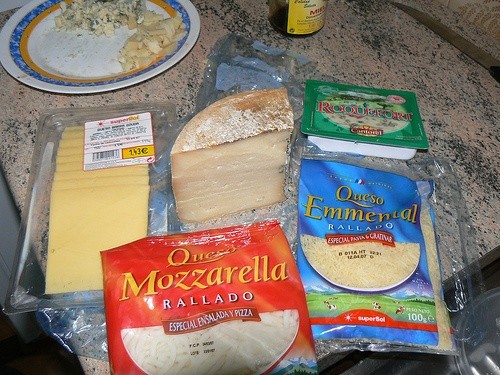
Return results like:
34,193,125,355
0,0,200,94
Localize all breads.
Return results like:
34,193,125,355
169,88,295,223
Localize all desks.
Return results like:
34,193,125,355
0,0,500,375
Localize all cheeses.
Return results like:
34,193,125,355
44,126,150,295
